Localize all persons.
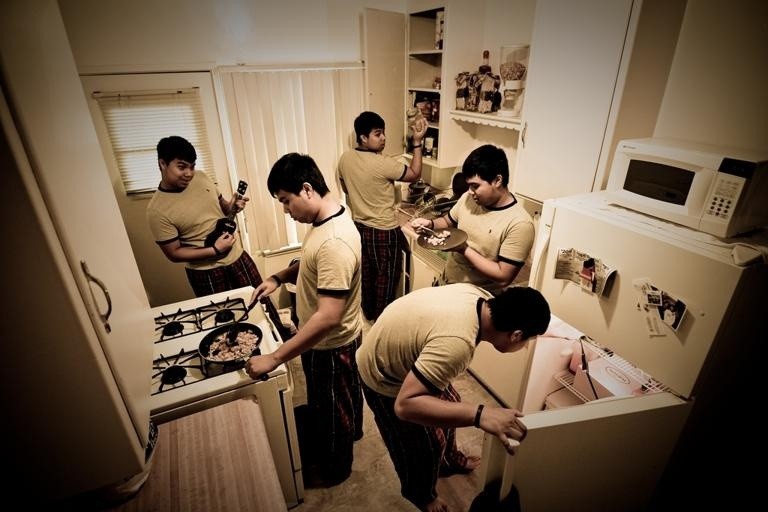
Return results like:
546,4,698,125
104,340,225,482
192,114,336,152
354,281,550,511
410,145,536,296
145,135,291,344
337,111,429,322
244,152,365,491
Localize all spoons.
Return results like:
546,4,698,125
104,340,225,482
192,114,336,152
414,222,447,238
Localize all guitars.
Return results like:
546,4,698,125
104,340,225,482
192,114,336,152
204,180,248,261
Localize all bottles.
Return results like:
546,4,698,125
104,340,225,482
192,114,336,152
454,50,500,118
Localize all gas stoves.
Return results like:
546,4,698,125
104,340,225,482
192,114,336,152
145,286,288,415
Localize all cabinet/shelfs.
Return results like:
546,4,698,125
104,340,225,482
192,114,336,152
511,1,686,209
402,157,455,189
364,5,461,170
451,3,534,191
0,1,158,498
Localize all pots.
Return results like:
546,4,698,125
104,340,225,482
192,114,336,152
409,179,431,204
198,322,269,381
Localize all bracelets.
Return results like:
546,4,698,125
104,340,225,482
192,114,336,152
270,275,281,287
211,245,221,256
430,219,435,229
474,402,483,429
413,145,423,148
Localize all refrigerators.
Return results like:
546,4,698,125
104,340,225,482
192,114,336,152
481,189,767,511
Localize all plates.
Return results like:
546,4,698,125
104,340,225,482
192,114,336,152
417,228,468,251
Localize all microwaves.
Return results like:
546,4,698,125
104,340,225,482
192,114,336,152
605,137,768,239
